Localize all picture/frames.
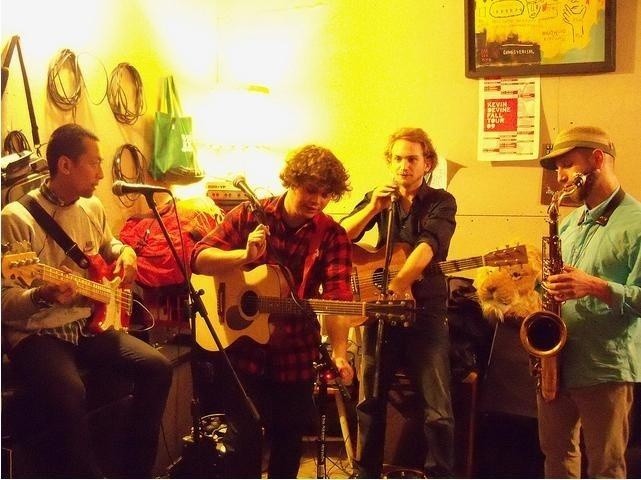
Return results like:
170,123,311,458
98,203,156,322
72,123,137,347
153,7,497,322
462,0,618,79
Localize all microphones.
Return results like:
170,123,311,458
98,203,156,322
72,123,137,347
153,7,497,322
391,183,400,202
112,181,168,196
233,175,263,209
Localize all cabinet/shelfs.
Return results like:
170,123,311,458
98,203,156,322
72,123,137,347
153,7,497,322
1,341,194,478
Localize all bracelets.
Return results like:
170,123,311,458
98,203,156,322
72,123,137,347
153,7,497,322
34,287,49,307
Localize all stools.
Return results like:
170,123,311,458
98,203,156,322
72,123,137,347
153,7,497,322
302,386,356,469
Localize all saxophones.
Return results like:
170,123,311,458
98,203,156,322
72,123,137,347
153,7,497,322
520,169,594,402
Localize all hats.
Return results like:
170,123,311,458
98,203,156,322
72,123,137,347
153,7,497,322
539,124,617,169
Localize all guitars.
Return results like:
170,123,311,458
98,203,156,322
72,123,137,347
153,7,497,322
1,240,133,335
188,264,414,352
319,243,528,328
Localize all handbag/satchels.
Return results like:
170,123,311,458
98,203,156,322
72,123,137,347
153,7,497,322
150,74,204,182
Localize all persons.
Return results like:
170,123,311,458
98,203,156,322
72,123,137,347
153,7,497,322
190,145,354,479
339,128,457,479
2,122,173,479
537,125,641,479
